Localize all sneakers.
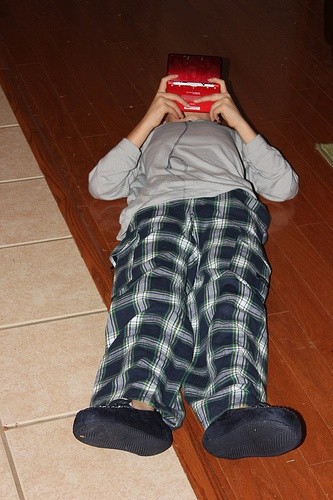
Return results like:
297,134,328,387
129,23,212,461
73,399,174,456
202,402,307,459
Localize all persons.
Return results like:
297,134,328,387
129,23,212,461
72,75,303,458
